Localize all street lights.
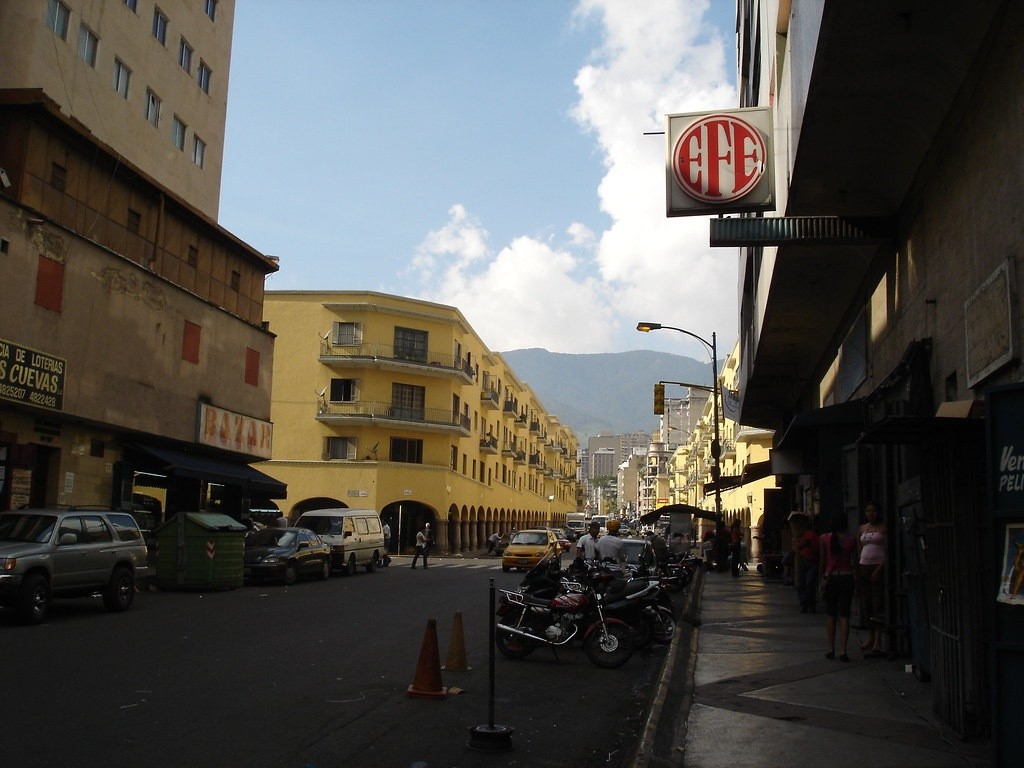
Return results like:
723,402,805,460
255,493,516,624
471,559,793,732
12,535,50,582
637,321,724,536
665,427,698,508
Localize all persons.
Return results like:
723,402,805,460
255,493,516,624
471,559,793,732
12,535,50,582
277,511,288,527
818,513,857,662
411,527,430,570
797,530,819,613
721,519,744,577
854,500,888,658
576,522,600,563
596,520,627,562
488,532,505,556
424,523,433,556
383,520,392,555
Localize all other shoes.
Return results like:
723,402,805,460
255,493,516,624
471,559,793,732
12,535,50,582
840,653,847,660
825,651,834,658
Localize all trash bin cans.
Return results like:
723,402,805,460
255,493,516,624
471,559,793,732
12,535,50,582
150,511,246,594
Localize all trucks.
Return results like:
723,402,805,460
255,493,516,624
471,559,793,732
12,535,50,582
565,512,586,540
591,515,609,538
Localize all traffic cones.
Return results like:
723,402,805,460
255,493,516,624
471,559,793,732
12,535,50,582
440,611,473,673
407,619,448,700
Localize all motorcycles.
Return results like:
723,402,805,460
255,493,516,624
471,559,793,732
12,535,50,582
752,535,795,586
495,551,707,668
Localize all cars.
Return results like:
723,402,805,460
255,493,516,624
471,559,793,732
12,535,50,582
244,527,332,585
618,522,632,536
493,527,517,556
530,526,572,552
621,538,657,575
502,530,561,573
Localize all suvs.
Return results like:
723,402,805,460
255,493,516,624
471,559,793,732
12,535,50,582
0,504,148,623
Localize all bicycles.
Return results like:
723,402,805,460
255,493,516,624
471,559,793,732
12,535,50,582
727,545,748,578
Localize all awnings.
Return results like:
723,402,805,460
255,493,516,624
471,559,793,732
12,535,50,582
772,395,865,449
114,431,287,499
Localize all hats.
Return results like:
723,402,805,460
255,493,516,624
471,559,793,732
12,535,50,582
607,520,621,531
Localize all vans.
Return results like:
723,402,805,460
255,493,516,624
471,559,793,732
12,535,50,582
292,508,385,578
